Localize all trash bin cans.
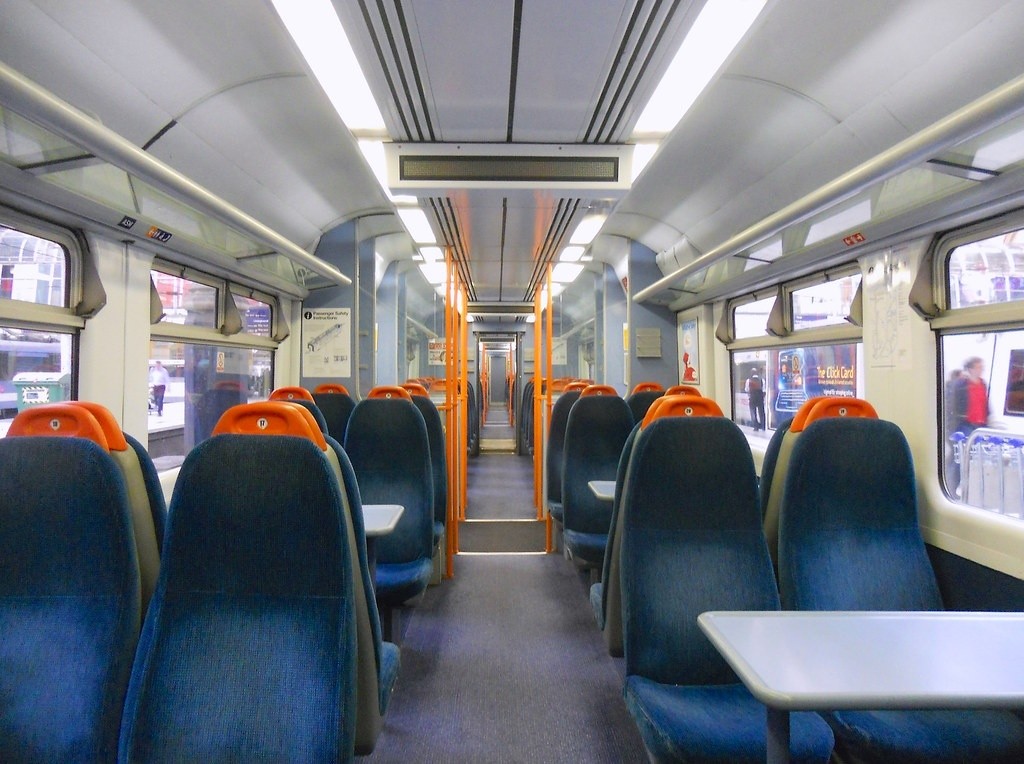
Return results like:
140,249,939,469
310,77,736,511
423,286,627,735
11,370,71,414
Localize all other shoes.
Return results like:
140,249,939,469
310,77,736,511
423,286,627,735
954,494,960,500
753,428,759,431
157,410,162,416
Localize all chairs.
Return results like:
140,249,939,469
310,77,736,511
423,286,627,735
0,373,1024,764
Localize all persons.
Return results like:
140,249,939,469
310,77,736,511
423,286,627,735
150,361,170,416
745,368,766,431
945,357,990,499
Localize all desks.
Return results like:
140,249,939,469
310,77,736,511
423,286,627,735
156,467,405,591
589,480,616,502
697,610,1024,764
